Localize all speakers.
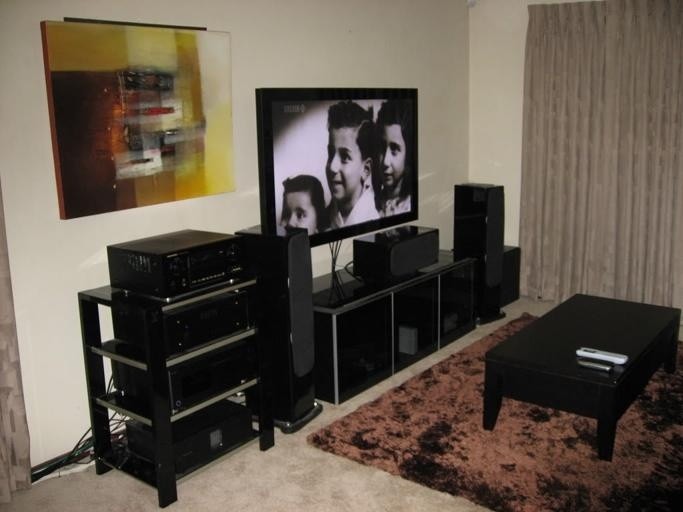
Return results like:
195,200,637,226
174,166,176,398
353,225,438,280
454,184,505,325
235,224,321,433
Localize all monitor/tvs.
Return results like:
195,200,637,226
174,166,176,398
256,88,418,248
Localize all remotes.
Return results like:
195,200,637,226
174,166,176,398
576,346,628,364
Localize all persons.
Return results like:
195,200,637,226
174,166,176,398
375,99,411,218
325,99,381,232
280,174,326,237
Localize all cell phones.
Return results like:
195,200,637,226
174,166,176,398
578,357,615,371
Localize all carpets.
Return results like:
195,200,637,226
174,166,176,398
306,311,683,512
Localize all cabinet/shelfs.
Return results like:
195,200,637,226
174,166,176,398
313,249,476,405
78,279,276,508
